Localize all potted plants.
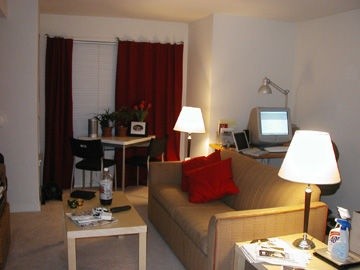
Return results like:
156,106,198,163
110,111,132,137
100,113,112,136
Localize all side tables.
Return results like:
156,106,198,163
235,232,360,270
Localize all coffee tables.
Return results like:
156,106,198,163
62,188,148,270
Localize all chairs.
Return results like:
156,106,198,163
125,134,170,189
71,137,118,192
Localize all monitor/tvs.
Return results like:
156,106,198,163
248,107,292,147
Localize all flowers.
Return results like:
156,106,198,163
134,100,152,121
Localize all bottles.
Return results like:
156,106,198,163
99,168,113,205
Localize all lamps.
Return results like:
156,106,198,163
278,129,342,251
173,105,206,158
259,76,290,107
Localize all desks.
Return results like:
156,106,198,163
210,144,286,158
77,134,155,192
147,148,329,270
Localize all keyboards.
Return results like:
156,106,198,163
264,147,288,153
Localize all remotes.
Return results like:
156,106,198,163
111,206,130,212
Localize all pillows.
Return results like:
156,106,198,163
181,150,221,192
184,157,240,203
